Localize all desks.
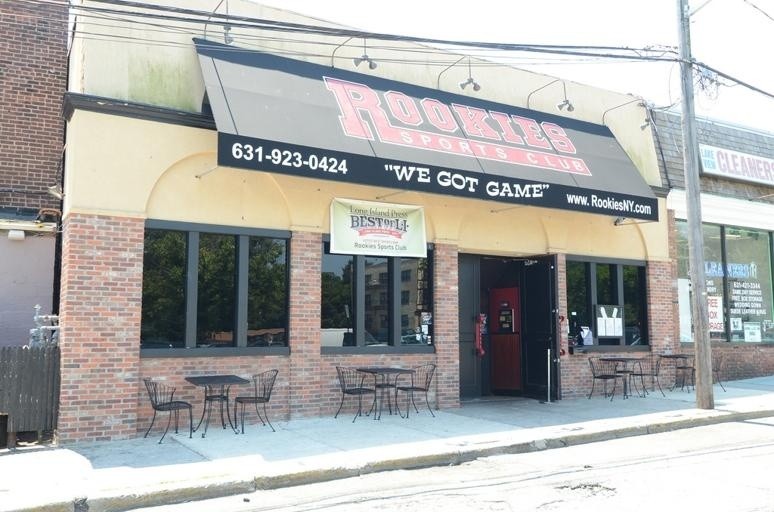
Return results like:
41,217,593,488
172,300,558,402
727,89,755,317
357,366,416,420
184,375,250,438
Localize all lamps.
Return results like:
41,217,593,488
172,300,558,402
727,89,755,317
460,80,482,90
48,143,68,198
354,56,377,70
223,29,233,44
558,100,574,112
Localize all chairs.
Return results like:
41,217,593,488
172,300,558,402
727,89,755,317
143,378,193,444
335,366,377,423
234,369,278,434
588,353,726,401
395,364,435,418
